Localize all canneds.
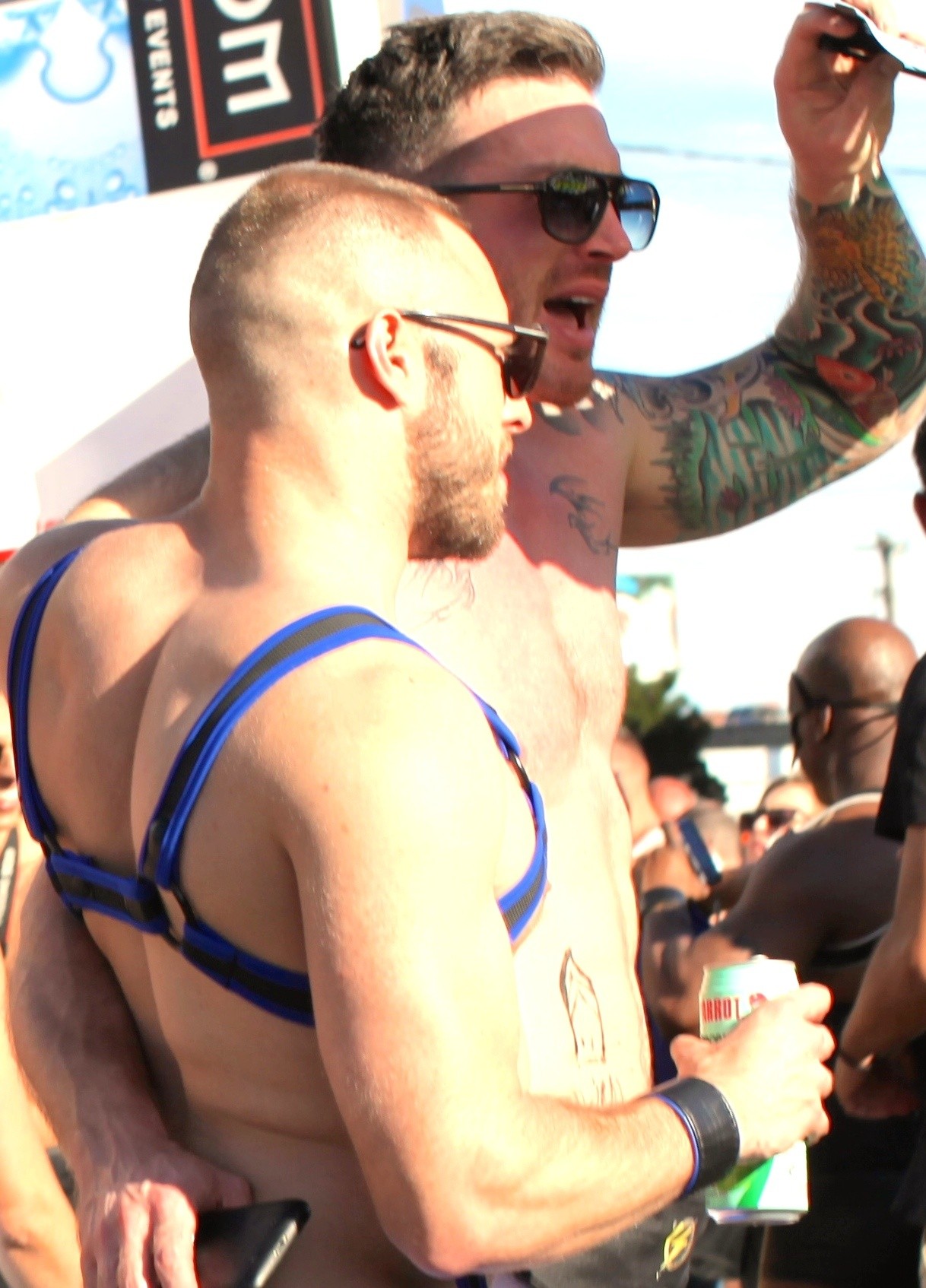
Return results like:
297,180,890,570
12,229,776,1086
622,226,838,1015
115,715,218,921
697,953,808,1225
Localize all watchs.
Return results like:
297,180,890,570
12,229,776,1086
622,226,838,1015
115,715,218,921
834,1040,916,1085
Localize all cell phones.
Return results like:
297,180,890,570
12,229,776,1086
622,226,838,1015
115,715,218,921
171,1196,309,1285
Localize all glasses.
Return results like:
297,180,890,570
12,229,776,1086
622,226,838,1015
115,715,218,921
351,310,551,398
429,167,662,252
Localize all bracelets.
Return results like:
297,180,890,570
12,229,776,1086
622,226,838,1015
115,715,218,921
638,885,691,918
642,1068,739,1201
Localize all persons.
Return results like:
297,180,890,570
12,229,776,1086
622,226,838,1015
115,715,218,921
638,611,925,1287
0,0,926,1285
608,724,826,910
0,157,835,1287
837,409,924,1116
